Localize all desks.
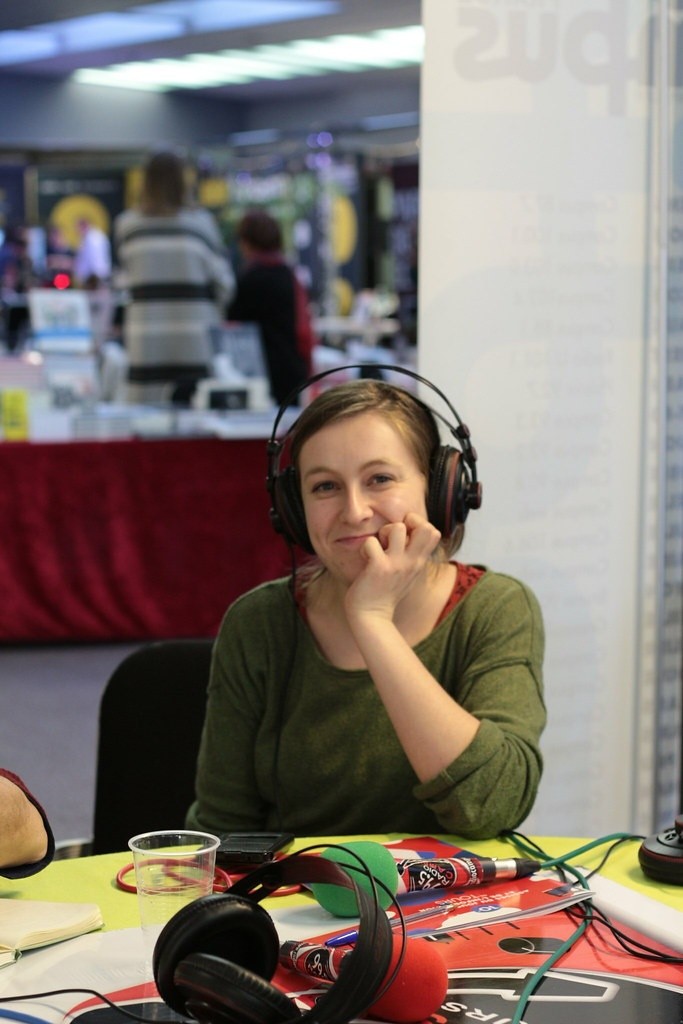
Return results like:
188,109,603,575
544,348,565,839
0,836,683,1024
0,435,310,643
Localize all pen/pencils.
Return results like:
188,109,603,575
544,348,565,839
324,904,457,947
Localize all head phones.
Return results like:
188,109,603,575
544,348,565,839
265,364,483,556
154,843,406,1024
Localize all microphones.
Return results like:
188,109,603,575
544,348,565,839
312,841,542,921
275,931,448,1023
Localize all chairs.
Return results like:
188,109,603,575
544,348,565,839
93,637,212,836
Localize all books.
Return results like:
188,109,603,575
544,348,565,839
0,898,105,971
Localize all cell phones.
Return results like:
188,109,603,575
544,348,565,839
194,831,295,864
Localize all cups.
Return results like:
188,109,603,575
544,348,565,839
128,831,222,941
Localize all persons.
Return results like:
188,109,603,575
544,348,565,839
221,215,314,408
0,214,112,358
114,152,237,411
179,380,549,846
0,766,48,870
